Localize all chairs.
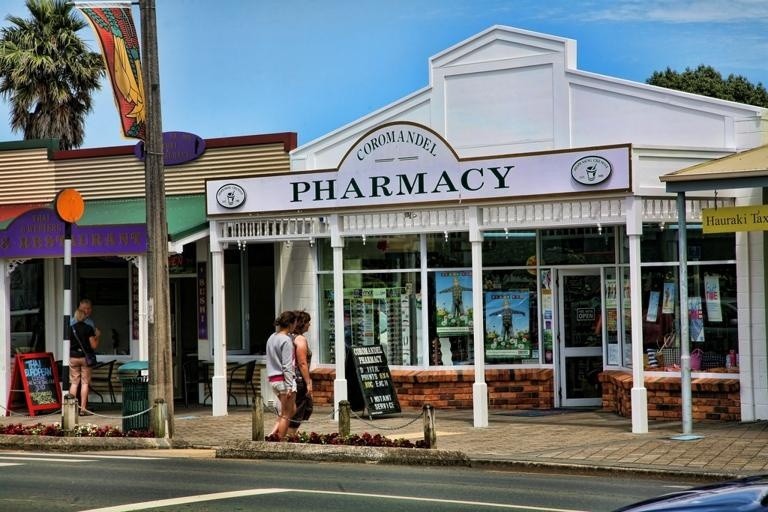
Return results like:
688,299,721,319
200,360,256,407
89,359,116,407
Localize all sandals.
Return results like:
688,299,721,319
79,411,94,417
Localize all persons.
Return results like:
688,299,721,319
69,298,96,336
286,311,313,435
439,277,472,317
68,306,100,416
488,300,526,342
265,310,299,438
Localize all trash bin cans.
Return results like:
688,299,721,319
122,377,148,430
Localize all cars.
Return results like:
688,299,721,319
10,308,40,356
692,296,738,346
610,475,768,512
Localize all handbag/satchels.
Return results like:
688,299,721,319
85,350,98,367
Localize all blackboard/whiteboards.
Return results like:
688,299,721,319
7,354,27,410
353,346,401,414
17,353,62,411
345,347,366,411
